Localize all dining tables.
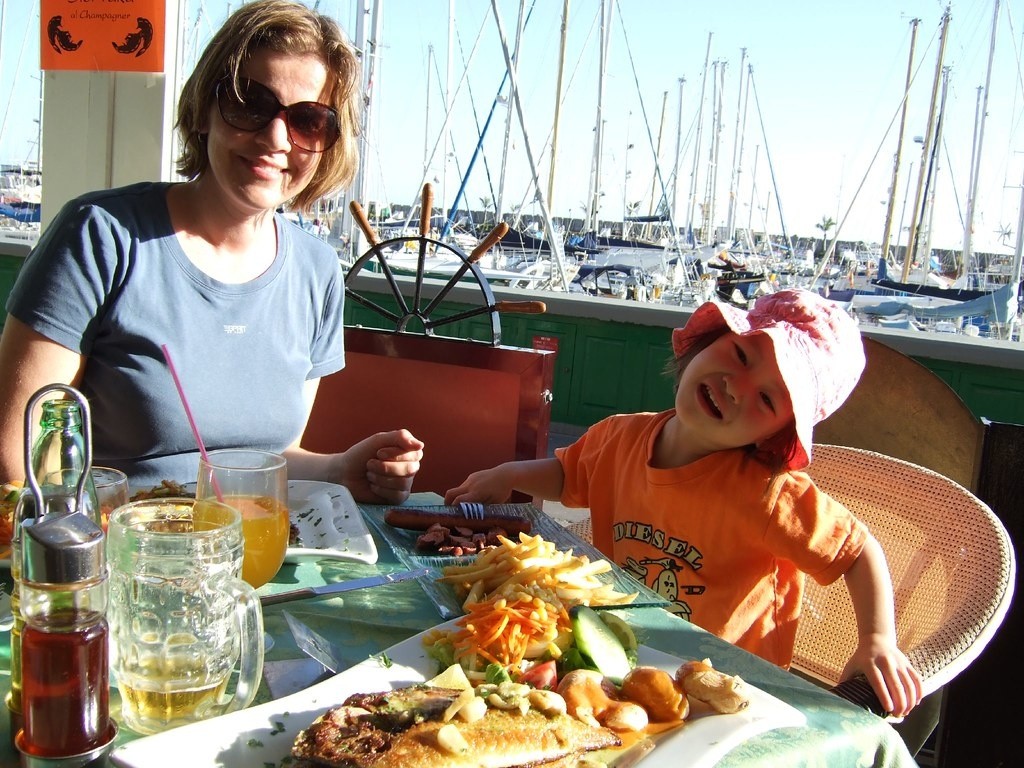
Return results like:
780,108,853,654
0,492,921,768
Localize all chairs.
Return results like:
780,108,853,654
566,442,1016,768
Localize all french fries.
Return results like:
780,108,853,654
435,532,640,610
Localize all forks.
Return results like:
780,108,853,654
461,502,484,520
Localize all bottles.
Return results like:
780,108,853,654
7,399,102,712
20,511,110,756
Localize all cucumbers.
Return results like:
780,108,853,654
571,604,630,679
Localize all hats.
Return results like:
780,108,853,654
673,288,869,477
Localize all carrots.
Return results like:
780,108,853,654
457,599,572,669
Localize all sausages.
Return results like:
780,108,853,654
386,508,531,537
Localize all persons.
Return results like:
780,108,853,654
0,0,423,507
444,286,925,719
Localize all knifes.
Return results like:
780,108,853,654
261,570,431,605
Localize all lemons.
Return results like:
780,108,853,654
600,612,636,652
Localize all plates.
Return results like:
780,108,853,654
0,485,7,572
355,502,672,616
125,480,378,563
109,615,806,768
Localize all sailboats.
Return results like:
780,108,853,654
0,0,1024,343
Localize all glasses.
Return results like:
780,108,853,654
214,74,341,153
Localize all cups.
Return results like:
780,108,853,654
46,467,128,543
105,499,266,735
196,453,290,590
